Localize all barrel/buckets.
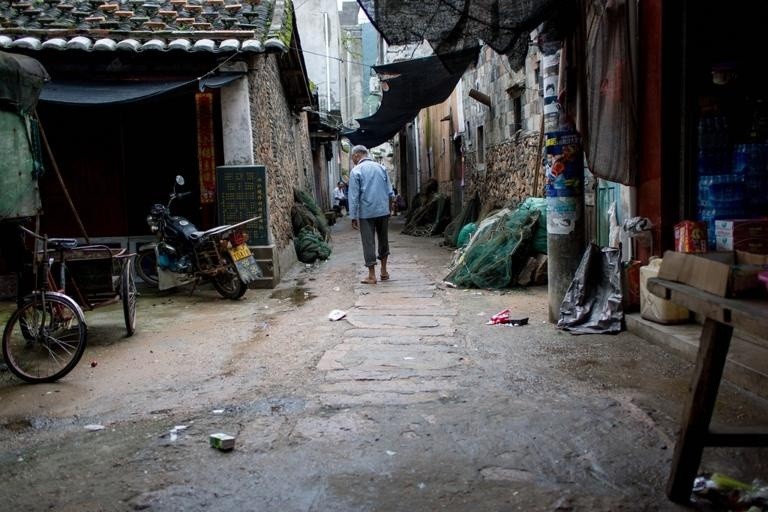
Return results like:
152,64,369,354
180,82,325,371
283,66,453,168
638,255,691,326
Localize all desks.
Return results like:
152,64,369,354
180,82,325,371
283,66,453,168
644,276,768,507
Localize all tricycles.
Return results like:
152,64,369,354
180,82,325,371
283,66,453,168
1,219,146,387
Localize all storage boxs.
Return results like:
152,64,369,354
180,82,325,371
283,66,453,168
671,218,766,251
658,248,762,298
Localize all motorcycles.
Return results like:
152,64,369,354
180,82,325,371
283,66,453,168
136,172,271,304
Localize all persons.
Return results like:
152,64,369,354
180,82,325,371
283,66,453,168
332,181,349,213
348,145,395,285
392,188,407,216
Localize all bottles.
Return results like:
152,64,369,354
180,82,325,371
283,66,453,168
698,116,768,250
551,154,570,177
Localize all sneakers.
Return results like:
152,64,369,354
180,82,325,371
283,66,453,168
360,277,378,285
380,272,390,280
